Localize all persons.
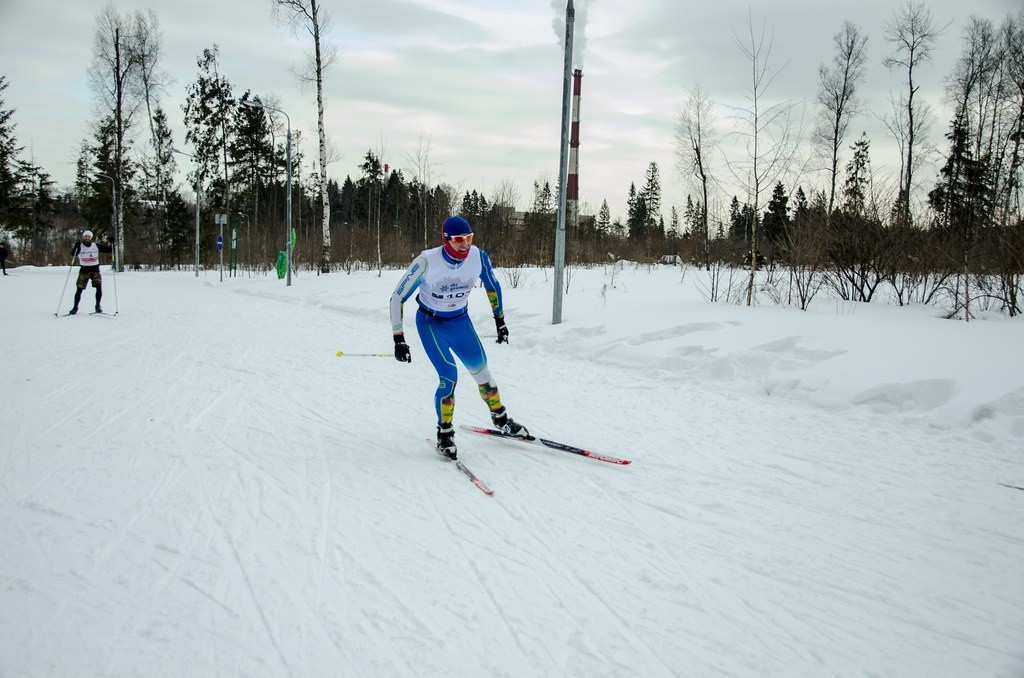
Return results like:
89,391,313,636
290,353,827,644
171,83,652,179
69,231,115,314
389,217,530,457
0,242,8,275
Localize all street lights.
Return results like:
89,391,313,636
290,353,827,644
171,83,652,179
241,100,292,286
91,171,119,273
171,149,200,278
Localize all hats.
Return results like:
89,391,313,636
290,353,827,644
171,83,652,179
443,216,472,240
83,230,94,238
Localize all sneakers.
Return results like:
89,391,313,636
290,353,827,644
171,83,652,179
436,431,456,457
70,309,77,314
96,307,102,312
494,414,524,435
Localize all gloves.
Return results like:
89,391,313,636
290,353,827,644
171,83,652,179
495,317,508,343
394,334,411,363
107,237,115,243
75,241,81,248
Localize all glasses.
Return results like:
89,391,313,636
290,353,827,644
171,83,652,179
449,232,473,243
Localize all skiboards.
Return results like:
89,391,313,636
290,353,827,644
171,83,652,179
425,423,633,495
55,311,121,319
